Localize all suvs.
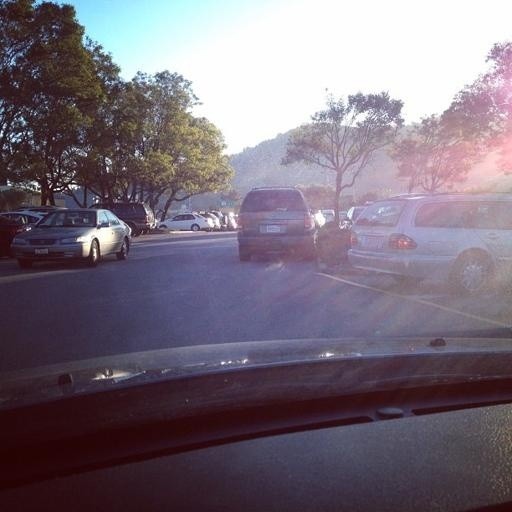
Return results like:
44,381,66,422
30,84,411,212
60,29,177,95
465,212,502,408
89,202,156,238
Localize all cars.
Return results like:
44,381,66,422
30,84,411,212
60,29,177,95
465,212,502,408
311,201,375,228
0,204,70,257
10,208,132,268
157,211,239,233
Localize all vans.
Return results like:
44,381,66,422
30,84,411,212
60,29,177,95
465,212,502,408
233,186,316,261
347,191,512,298
